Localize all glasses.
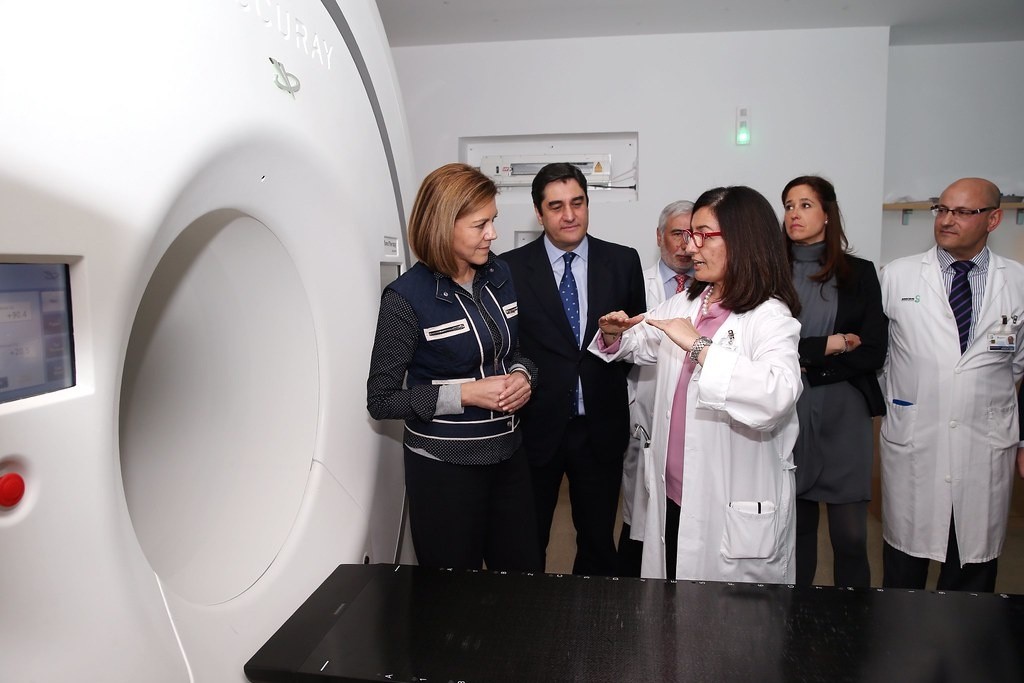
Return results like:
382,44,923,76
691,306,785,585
681,229,727,248
931,205,995,219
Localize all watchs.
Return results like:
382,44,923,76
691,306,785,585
689,336,713,364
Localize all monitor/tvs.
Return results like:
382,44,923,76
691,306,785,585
0,262,76,404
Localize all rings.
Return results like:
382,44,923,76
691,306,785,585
522,396,525,401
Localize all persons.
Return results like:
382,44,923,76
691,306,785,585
782,176,890,588
584,185,804,583
615,201,696,577
498,162,646,576
367,162,539,572
877,178,1024,595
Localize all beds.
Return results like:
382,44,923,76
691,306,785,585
246,564,1024,683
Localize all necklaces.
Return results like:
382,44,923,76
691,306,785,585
702,285,713,316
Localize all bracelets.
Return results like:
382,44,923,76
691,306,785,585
839,333,848,354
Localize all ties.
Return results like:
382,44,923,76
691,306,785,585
949,260,972,355
556,252,580,420
673,274,690,294
